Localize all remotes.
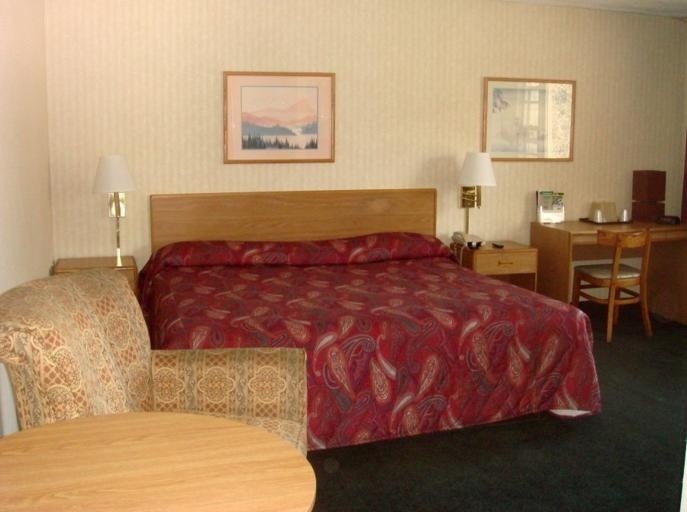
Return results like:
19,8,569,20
491,241,504,248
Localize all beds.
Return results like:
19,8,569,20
148,187,588,453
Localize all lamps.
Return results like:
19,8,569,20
457,152,496,209
91,154,135,267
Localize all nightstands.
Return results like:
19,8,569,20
50,256,139,297
449,239,538,293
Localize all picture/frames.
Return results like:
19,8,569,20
222,70,335,165
481,76,576,163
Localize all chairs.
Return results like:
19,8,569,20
0,270,310,459
571,227,651,343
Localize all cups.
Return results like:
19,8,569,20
619,208,630,223
595,209,603,222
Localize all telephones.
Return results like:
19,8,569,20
452,231,482,246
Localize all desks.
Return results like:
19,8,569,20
529,217,686,325
0,411,318,512
